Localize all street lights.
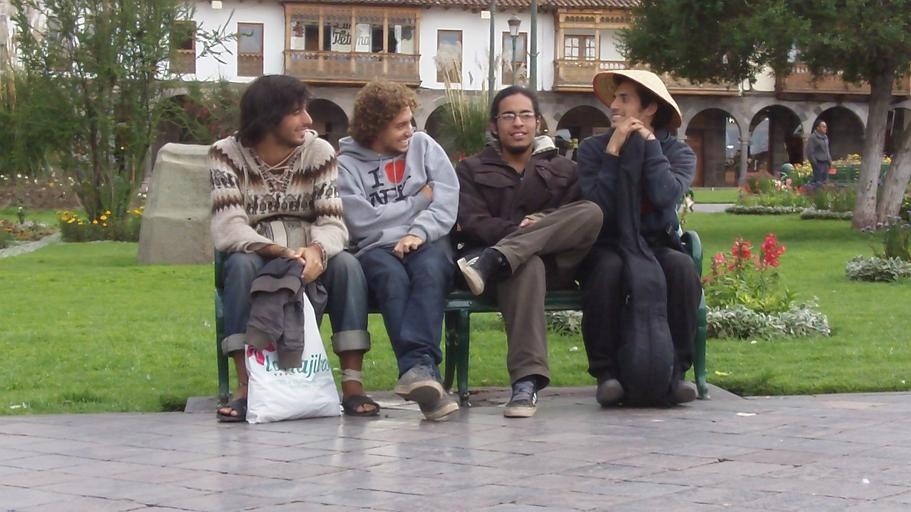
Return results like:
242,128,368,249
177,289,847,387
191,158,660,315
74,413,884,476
506,11,521,87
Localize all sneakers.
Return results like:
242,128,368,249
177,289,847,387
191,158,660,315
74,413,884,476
419,389,460,421
504,379,540,418
456,248,508,297
666,381,697,403
393,364,444,404
597,367,626,406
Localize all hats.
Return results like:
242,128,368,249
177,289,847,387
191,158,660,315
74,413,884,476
593,69,682,131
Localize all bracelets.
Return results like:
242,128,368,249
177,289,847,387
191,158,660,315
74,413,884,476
643,132,653,139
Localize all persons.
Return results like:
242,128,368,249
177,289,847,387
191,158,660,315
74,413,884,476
453,86,605,418
336,76,460,422
806,121,833,208
576,69,701,406
207,76,379,422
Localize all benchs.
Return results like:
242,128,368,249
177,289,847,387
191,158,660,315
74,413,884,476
215,229,712,406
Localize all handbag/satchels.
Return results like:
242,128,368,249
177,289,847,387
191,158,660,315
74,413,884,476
245,293,341,425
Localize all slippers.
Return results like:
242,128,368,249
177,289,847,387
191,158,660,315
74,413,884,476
216,397,247,422
342,394,380,417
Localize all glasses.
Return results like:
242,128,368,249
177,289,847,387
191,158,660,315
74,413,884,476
494,112,536,123
